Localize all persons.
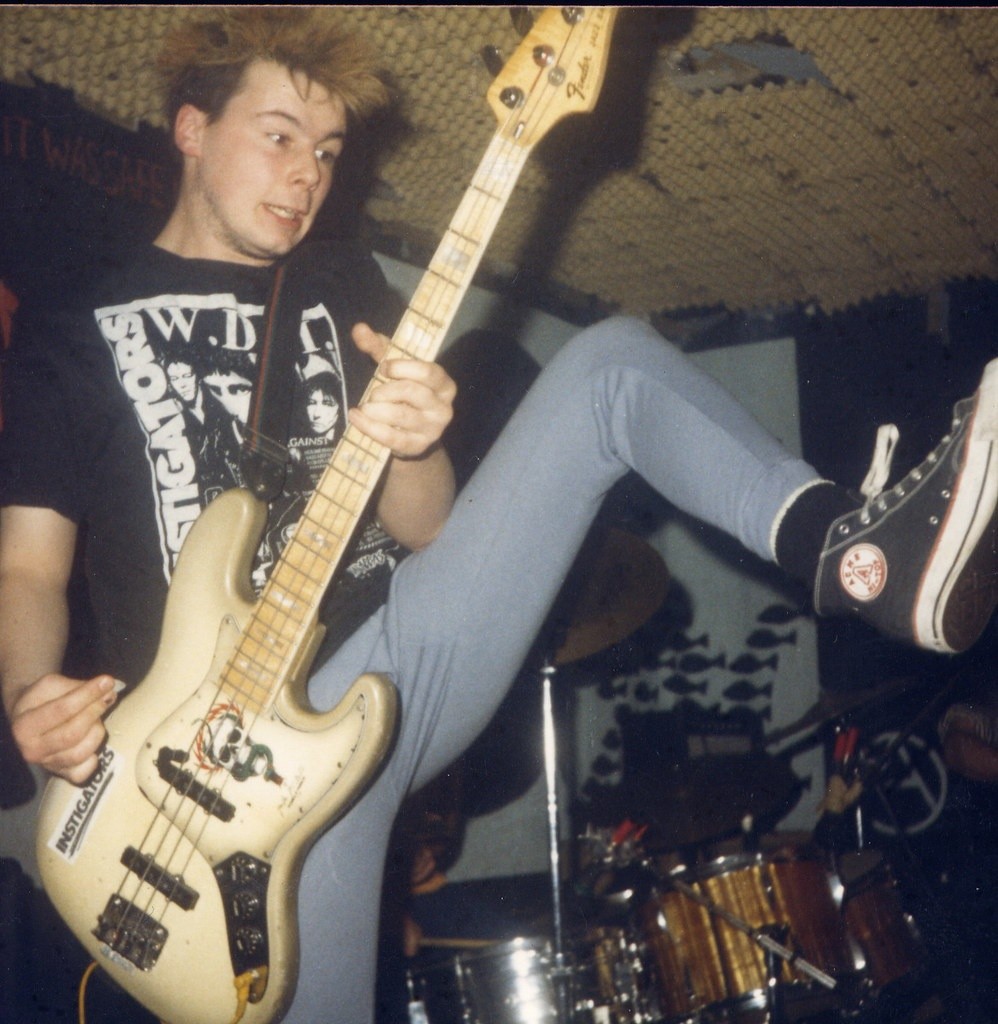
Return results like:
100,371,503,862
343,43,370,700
1,1,997,1024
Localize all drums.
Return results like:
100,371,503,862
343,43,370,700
823,843,935,1016
632,848,853,1024
405,922,666,1024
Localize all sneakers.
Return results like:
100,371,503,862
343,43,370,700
814,359,997,655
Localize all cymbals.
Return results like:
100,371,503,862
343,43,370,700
525,519,667,664
755,673,910,746
590,752,798,847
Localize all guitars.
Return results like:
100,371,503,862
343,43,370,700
37,6,618,1024
